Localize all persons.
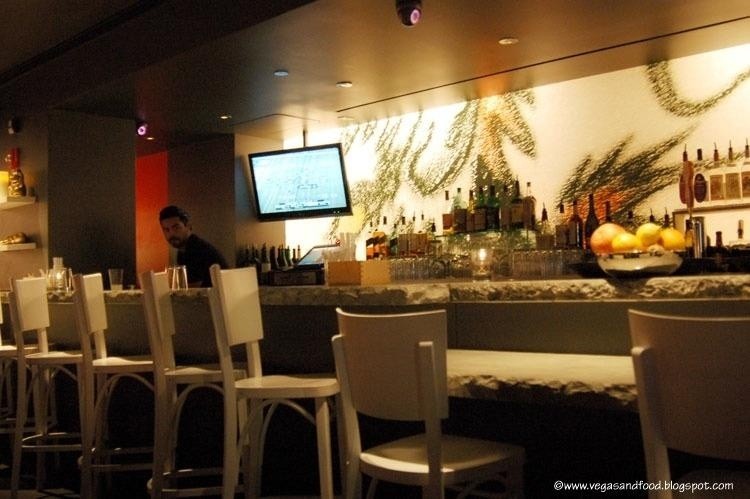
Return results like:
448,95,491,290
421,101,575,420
158,205,229,287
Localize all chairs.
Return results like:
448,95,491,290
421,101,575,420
626,307,750,499
0,264,528,499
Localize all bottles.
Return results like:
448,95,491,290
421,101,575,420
536,192,672,249
234,243,301,285
365,212,438,259
678,137,750,204
441,174,539,232
686,216,750,273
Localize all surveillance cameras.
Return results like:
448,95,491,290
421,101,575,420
136,120,149,136
395,0,423,27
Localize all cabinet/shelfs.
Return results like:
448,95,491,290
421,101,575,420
0,195,39,251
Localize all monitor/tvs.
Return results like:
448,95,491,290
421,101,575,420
247,142,354,223
296,245,338,268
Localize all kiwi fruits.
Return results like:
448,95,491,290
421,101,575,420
647,243,664,254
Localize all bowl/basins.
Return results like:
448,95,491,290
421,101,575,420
596,250,684,283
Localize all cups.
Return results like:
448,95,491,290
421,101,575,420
510,249,583,279
108,268,125,291
389,258,433,281
163,264,189,289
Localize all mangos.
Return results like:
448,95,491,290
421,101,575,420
635,222,663,245
656,228,688,251
611,232,643,253
